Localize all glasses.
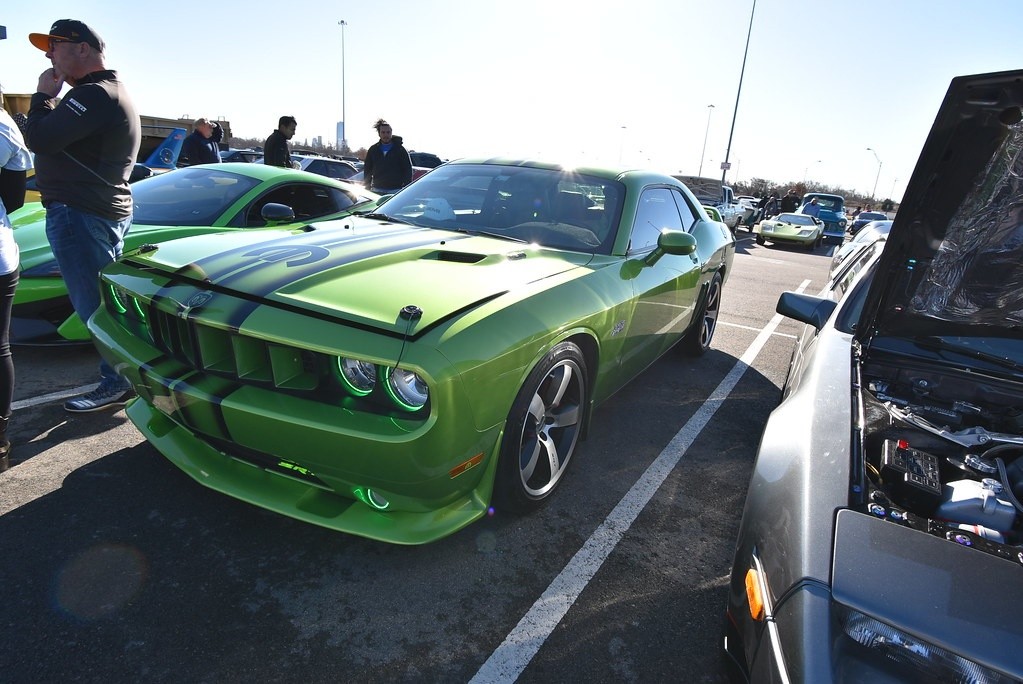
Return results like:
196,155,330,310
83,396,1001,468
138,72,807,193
48,38,81,52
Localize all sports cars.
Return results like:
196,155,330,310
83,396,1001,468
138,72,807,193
86,158,735,549
7,162,382,342
756,213,824,252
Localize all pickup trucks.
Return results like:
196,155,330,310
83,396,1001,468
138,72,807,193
671,175,746,235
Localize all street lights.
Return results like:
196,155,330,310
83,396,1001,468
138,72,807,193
338,20,349,151
867,148,882,205
699,105,715,176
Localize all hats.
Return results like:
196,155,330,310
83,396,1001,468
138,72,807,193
28,19,105,53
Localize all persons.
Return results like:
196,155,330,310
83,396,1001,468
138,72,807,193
758,189,800,223
175,117,224,167
846,206,861,232
24,19,142,411
0,107,35,471
364,118,413,194
865,204,871,212
802,196,820,218
264,116,297,168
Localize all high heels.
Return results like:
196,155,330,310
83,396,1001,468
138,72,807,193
0,440,11,472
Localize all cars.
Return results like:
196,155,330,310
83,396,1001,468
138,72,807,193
738,195,784,224
220,150,443,198
851,211,889,234
796,192,848,245
722,72,1023,684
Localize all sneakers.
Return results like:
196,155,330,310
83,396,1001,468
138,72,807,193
64,377,140,413
